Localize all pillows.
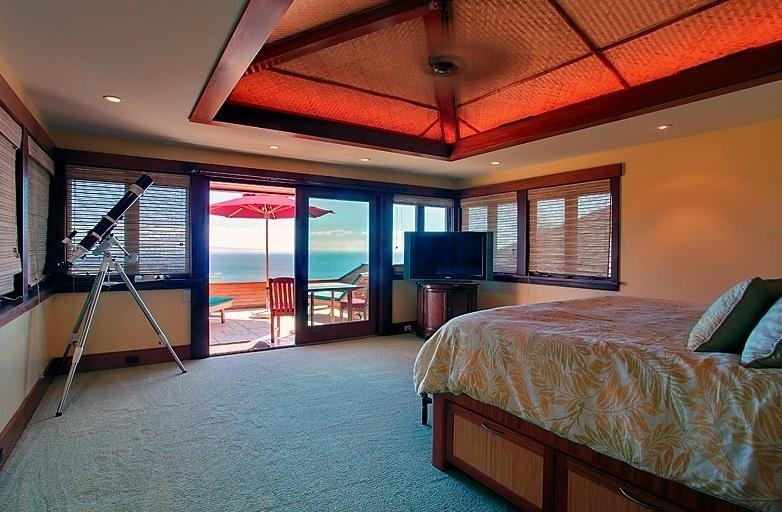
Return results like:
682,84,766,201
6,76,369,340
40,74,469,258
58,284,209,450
684,275,781,373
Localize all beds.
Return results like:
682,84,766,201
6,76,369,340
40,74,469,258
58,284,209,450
424,294,782,510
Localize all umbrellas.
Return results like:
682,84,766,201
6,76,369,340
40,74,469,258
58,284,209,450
209,191,335,309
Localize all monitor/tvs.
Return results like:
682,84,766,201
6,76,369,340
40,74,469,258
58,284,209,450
404,230,494,284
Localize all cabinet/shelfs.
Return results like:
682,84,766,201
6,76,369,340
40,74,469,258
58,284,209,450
413,278,480,340
443,398,679,512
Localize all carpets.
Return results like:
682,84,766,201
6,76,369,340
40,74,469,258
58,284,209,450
209,319,272,346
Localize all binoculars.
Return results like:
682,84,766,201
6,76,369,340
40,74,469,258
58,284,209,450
56,174,155,274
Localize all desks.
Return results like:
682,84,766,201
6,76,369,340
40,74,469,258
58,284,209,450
300,281,364,326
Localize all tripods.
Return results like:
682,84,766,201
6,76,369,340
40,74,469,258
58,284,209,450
56,241,186,418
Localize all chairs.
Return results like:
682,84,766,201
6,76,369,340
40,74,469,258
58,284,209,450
268,276,296,341
209,294,234,324
309,272,368,322
